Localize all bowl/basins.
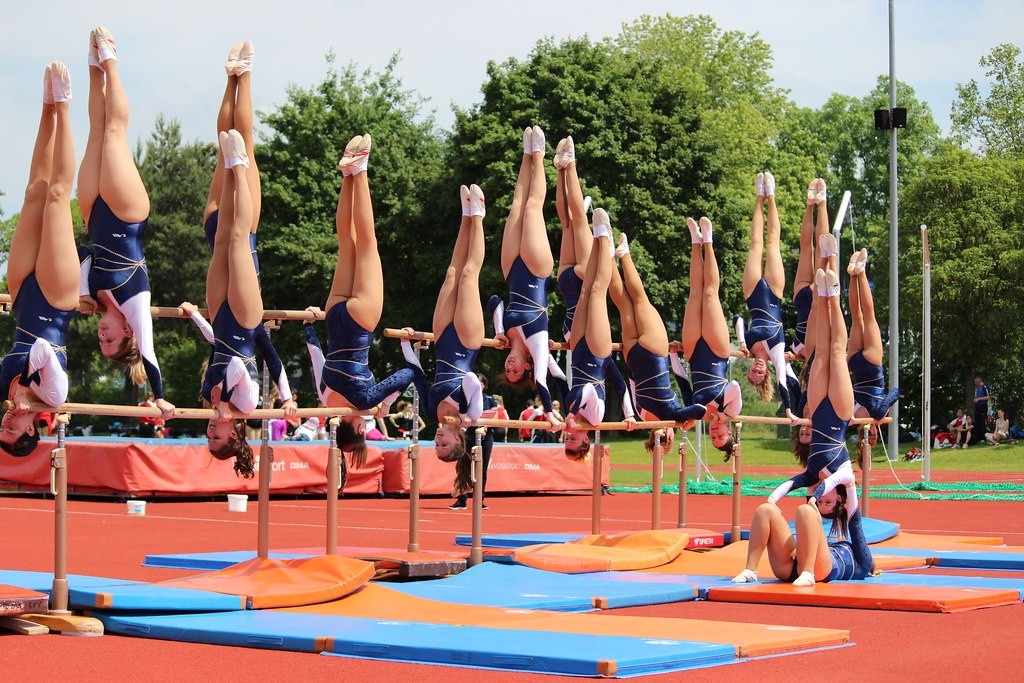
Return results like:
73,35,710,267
314,430,329,440
127,500,146,515
227,494,248,511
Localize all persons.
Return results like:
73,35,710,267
845,248,901,471
76,27,176,421
235,387,327,437
0,62,82,457
302,134,416,468
400,184,483,462
766,269,858,539
177,39,299,480
362,416,387,440
782,233,839,469
137,392,168,439
731,170,830,400
731,504,882,586
487,121,615,462
668,216,743,461
492,396,566,442
947,377,1024,449
608,232,706,459
390,399,429,440
448,372,497,510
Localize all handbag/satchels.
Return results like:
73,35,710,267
934,432,955,449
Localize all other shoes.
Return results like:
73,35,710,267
953,440,1004,449
225,42,244,77
227,129,250,168
88,31,105,73
52,63,72,103
43,65,56,105
234,40,254,76
554,138,567,169
469,184,486,219
448,502,467,510
339,135,363,176
531,126,545,157
93,26,117,64
559,135,575,168
792,571,815,586
522,127,532,155
687,172,826,246
218,131,233,168
460,185,471,217
593,209,629,258
731,569,757,583
482,504,489,510
815,234,868,297
347,133,373,177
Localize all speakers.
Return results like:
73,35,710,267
874,109,890,130
892,108,906,128
